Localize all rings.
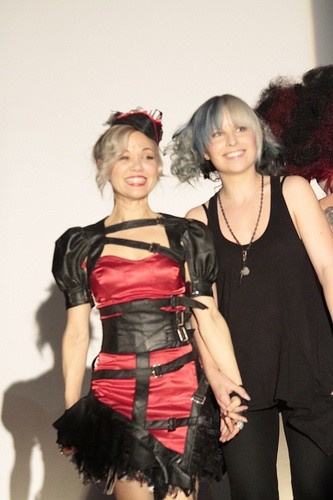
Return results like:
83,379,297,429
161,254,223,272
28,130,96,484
236,421,245,431
58,448,64,455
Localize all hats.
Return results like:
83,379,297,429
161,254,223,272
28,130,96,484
104,106,164,144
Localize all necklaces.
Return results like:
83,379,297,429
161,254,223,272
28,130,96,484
218,176,265,283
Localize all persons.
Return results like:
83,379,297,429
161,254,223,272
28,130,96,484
255,63,333,231
164,94,333,500
52,106,250,500
